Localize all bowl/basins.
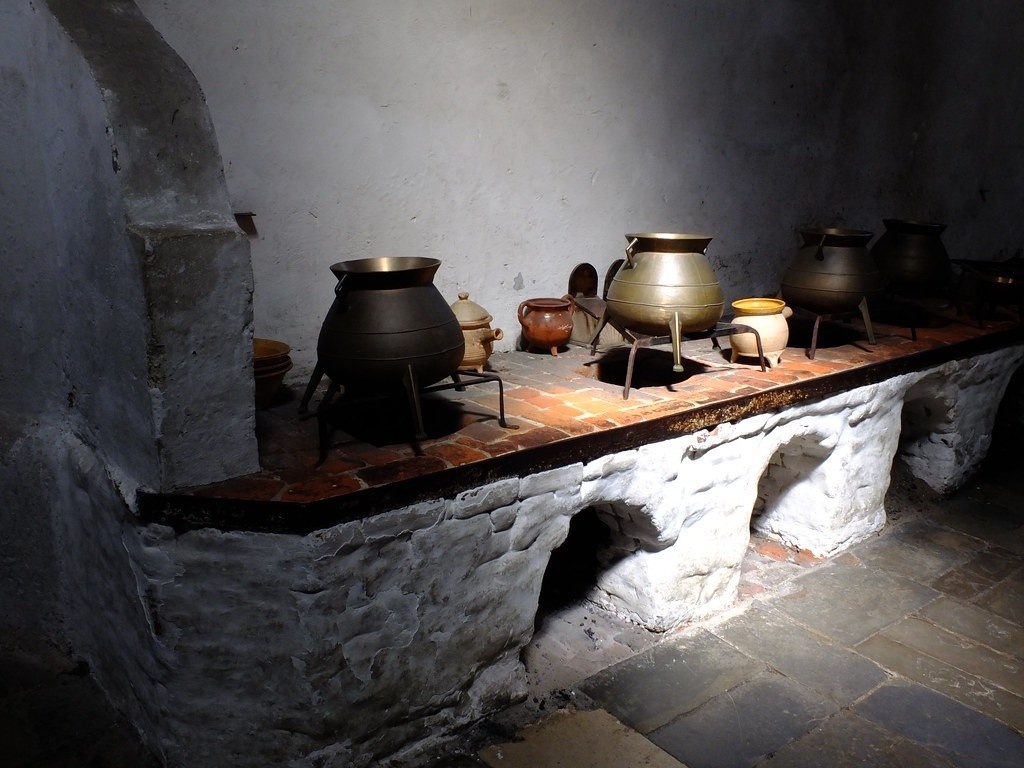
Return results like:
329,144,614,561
252,337,293,397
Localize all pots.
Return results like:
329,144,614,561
729,297,794,366
606,232,725,336
780,228,882,316
874,218,949,299
516,294,576,359
449,291,506,374
316,255,465,392
